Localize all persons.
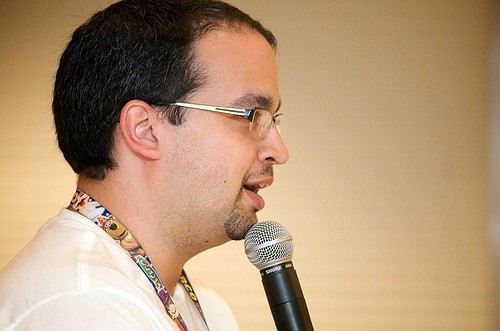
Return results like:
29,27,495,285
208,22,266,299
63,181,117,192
0,0,289,331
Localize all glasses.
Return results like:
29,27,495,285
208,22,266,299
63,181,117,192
170,102,284,138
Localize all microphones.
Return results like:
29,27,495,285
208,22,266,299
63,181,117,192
244,220,314,331
